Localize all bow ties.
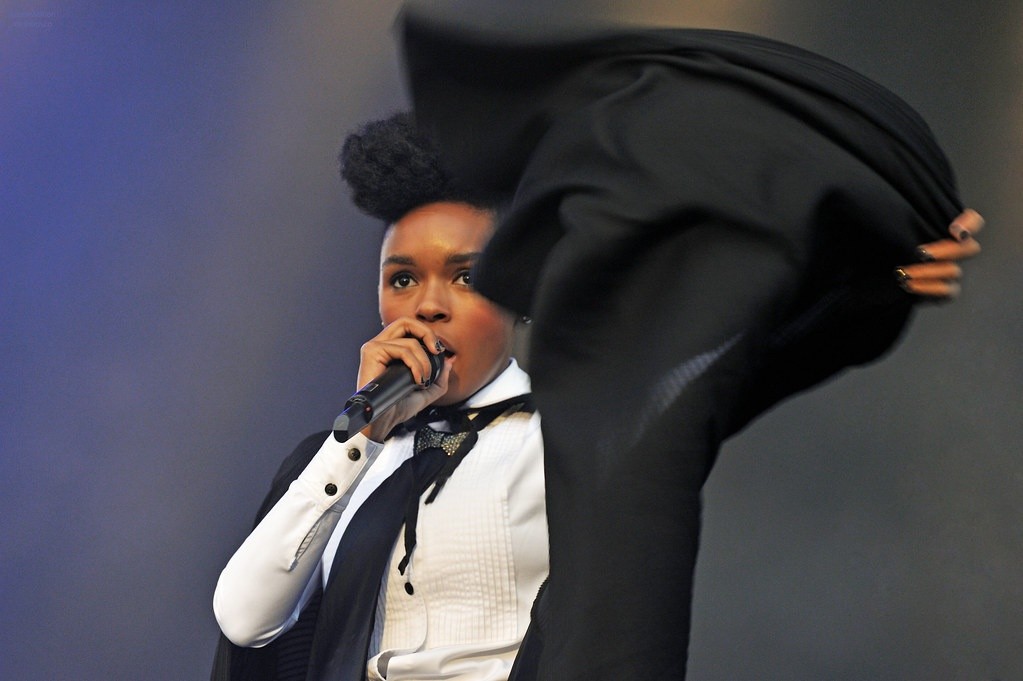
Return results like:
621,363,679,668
417,425,467,455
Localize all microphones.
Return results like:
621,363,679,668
333,342,446,444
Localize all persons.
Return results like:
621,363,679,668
203,104,986,681
